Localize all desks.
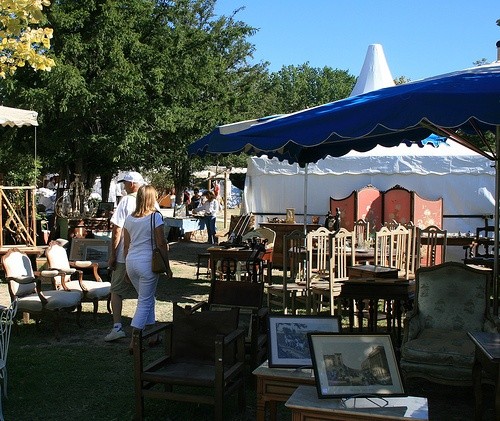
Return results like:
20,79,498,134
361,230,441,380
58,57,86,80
208,246,271,285
261,220,321,257
413,231,486,267
290,247,398,266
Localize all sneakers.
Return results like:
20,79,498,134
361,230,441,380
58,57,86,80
104,328,126,342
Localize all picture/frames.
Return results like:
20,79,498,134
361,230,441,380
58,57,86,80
308,330,408,398
266,313,343,369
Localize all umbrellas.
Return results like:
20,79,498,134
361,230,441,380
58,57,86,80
186,60,500,314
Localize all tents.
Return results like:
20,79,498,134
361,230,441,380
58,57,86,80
241,44,497,265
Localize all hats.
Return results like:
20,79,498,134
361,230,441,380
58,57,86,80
117,172,144,184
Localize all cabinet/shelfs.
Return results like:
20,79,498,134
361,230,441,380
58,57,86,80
253,360,316,421
285,384,429,421
339,282,410,341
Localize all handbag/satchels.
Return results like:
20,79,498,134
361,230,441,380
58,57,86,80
150,212,166,274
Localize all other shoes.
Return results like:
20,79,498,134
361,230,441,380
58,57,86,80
214,242,218,245
205,241,212,244
128,345,133,356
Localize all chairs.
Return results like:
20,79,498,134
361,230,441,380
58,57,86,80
0,217,500,421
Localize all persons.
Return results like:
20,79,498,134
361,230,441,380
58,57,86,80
193,190,219,244
189,188,201,210
124,184,173,352
103,172,160,341
